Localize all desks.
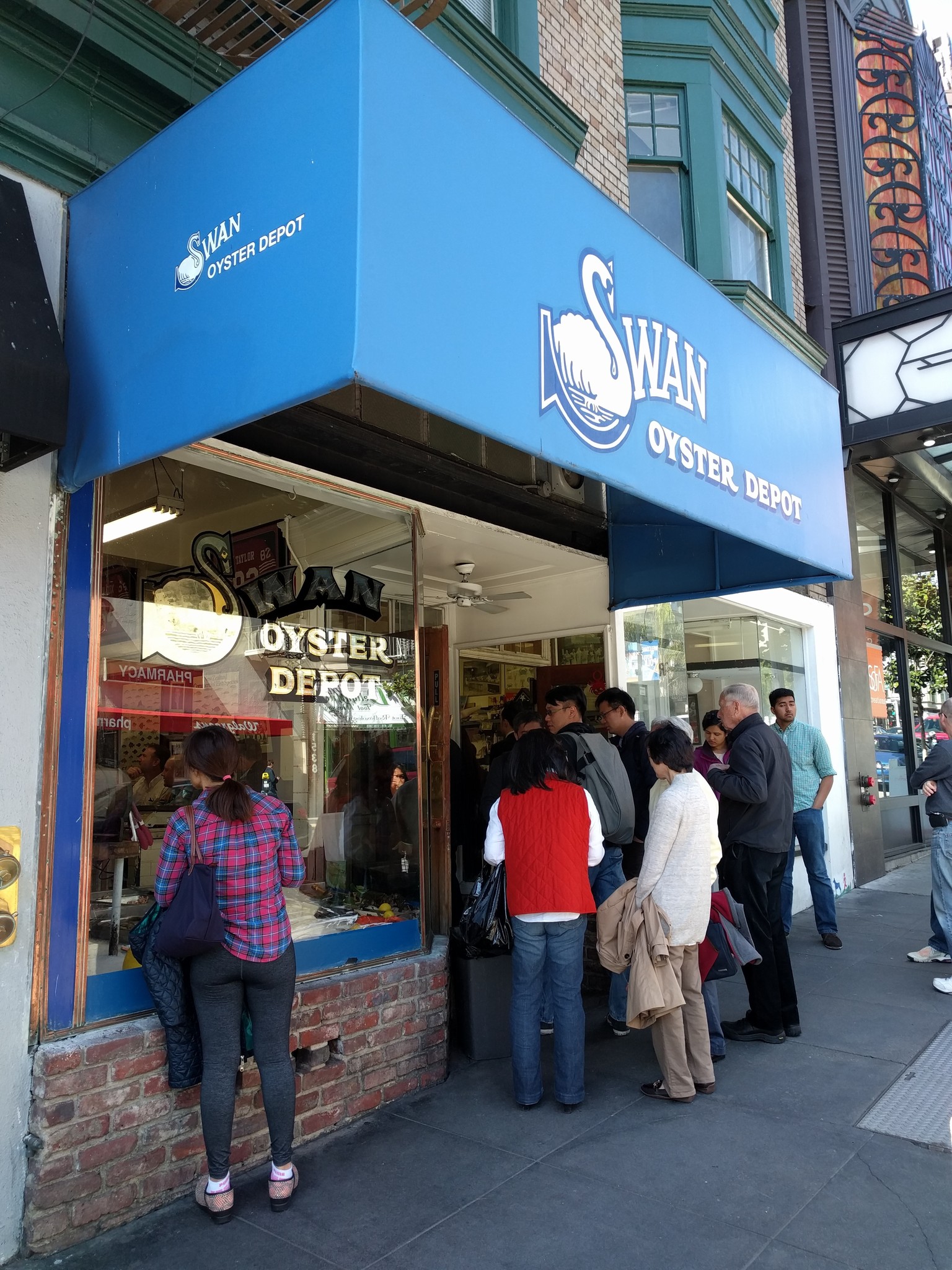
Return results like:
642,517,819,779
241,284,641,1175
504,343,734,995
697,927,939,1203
460,715,505,770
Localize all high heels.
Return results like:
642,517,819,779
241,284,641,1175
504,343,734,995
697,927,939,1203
563,1102,582,1115
521,1101,533,1111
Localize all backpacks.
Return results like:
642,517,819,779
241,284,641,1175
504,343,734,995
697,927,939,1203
558,732,635,843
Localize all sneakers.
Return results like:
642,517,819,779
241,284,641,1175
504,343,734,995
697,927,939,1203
906,945,951,963
932,974,952,995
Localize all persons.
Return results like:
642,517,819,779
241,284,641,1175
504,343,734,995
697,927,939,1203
691,709,731,803
767,688,842,950
648,716,727,1064
154,719,307,1223
261,758,280,800
907,697,952,994
635,717,717,1105
126,745,172,808
160,754,202,808
483,727,606,1114
708,682,802,1045
392,764,409,796
305,683,658,1036
235,737,266,793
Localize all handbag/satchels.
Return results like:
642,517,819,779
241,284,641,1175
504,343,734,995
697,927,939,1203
152,805,226,960
449,859,515,960
702,919,738,982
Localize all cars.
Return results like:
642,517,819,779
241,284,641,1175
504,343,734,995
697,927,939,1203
874,733,932,785
873,714,949,747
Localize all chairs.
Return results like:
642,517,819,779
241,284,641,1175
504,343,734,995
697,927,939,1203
889,741,898,752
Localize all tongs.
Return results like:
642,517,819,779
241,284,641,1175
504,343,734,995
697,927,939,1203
129,801,154,850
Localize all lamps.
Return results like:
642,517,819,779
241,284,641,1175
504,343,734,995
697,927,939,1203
935,512,946,519
922,434,936,447
687,672,704,694
102,457,188,544
924,543,935,554
663,618,732,635
887,476,899,483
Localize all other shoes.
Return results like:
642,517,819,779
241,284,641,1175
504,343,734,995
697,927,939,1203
195,1174,234,1224
822,932,842,949
268,1162,298,1212
722,1018,786,1043
607,1012,631,1036
539,1020,553,1035
784,1021,803,1037
641,1080,695,1103
695,1080,715,1094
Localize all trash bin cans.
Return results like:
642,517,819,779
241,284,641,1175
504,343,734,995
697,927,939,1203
452,942,513,1061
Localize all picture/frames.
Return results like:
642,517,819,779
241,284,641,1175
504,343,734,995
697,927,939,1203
462,661,502,697
669,694,702,745
168,738,192,783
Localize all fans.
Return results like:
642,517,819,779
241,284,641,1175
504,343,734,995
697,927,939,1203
393,562,533,615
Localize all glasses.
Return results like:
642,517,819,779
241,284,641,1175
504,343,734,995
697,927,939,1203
599,707,626,720
547,706,577,716
